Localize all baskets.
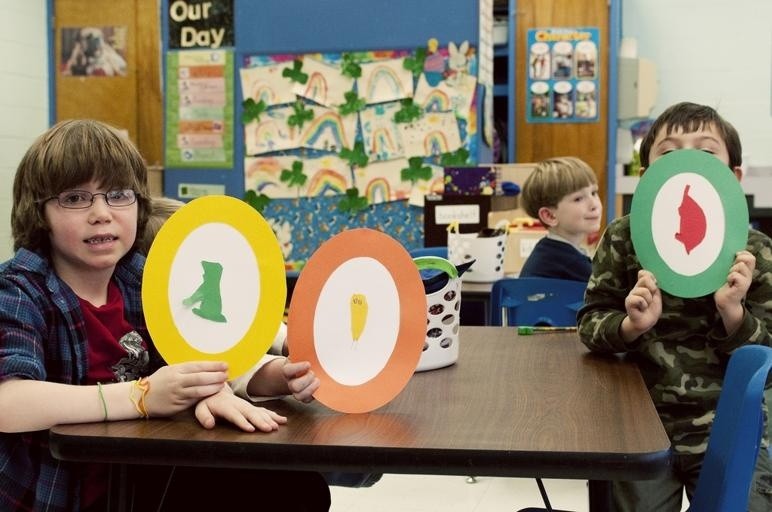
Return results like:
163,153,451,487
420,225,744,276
409,256,462,374
446,219,510,284
491,195,517,211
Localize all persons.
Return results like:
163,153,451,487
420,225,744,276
130,197,321,404
1,119,332,510
578,102,771,510
518,156,603,326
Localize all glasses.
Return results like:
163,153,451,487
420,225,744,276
43,190,140,209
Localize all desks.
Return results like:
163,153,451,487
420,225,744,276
461,282,495,326
48,325,673,512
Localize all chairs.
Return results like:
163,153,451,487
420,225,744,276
491,279,588,330
519,342,771,511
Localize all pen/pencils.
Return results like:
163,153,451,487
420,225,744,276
517,326,578,335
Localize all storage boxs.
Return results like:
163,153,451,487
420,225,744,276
423,192,518,248
502,227,551,276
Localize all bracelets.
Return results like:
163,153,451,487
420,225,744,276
94,378,109,419
130,376,151,418
